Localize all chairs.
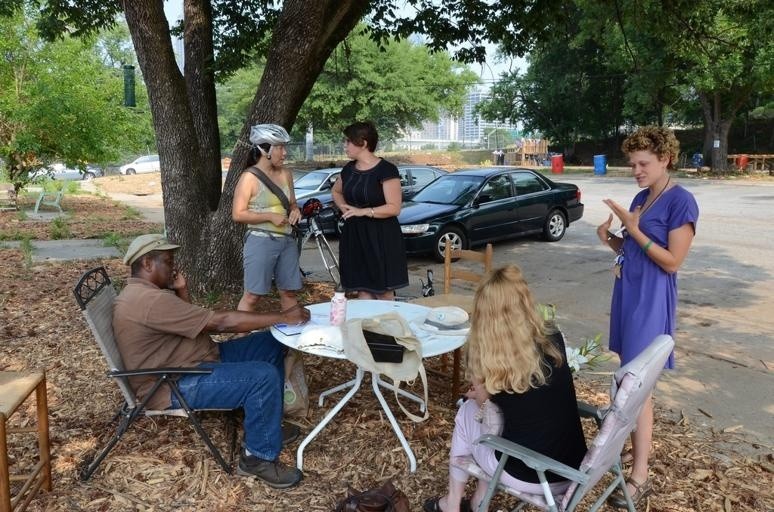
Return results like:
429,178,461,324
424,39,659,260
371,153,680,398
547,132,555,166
410,240,493,406
0,371,52,511
73,267,239,480
449,334,675,511
33,192,63,215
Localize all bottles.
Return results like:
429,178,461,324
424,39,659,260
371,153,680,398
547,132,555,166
328,287,347,324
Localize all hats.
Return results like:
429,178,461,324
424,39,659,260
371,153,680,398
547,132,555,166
123,233,182,266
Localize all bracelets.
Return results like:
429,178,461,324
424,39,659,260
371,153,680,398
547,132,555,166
642,239,653,253
369,206,374,217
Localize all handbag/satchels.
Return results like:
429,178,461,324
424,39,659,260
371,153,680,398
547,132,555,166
343,313,423,384
282,351,312,419
334,479,412,512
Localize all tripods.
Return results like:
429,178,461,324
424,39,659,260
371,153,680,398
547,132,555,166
301,218,341,287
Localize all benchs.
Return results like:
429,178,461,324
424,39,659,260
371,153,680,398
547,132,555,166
0,199,17,213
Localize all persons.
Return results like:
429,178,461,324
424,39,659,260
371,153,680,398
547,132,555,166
422,264,588,512
491,147,504,165
594,126,702,508
111,231,309,490
232,121,303,338
330,121,408,301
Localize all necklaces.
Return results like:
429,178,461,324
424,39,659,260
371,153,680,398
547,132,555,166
639,176,671,217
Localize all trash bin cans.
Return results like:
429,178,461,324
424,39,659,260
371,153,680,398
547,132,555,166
494,153,504,165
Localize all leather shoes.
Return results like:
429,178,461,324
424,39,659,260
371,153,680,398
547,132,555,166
621,448,657,468
423,495,476,512
606,476,654,510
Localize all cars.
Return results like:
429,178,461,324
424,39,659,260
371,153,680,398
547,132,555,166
393,167,584,263
28,163,104,181
295,164,450,237
293,167,342,202
120,155,161,175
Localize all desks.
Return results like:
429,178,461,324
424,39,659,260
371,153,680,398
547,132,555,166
269,298,470,475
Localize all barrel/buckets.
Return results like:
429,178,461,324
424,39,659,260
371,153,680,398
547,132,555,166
593,155,607,175
552,154,564,173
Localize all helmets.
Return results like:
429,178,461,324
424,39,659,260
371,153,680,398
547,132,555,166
249,124,293,146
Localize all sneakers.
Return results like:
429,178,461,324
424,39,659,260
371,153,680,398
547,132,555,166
238,447,303,489
240,423,299,449
